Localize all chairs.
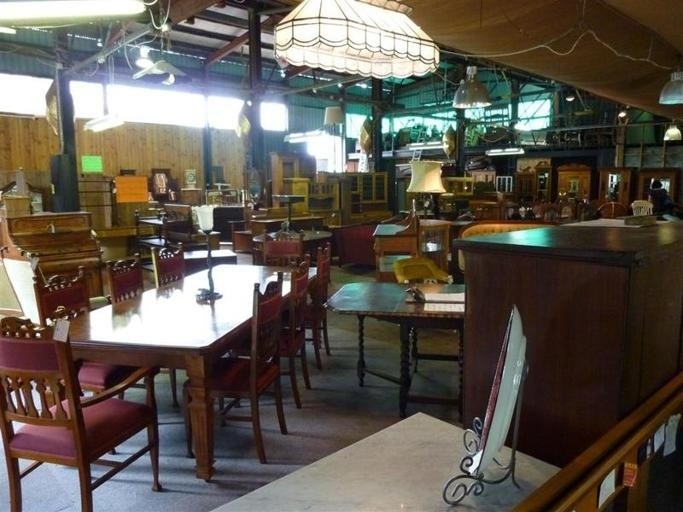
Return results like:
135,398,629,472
0,187,594,511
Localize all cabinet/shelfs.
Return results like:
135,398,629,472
242,147,683,227
449,218,683,469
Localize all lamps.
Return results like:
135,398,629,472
0,0,147,29
657,69,683,105
662,119,682,142
271,0,441,80
402,161,446,218
322,106,346,173
451,58,491,109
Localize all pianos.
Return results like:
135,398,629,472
0,212,104,326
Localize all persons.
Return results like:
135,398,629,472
648,180,667,216
